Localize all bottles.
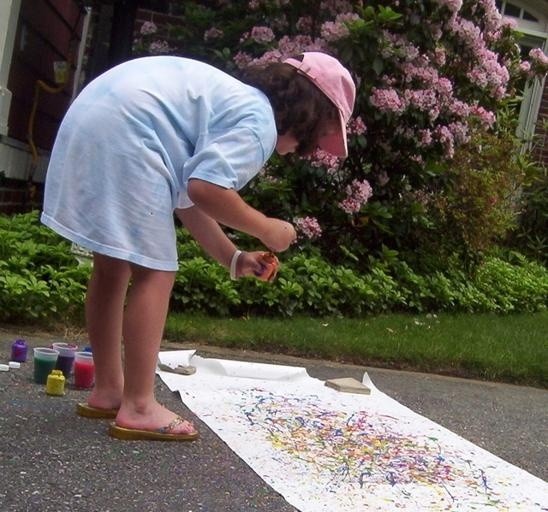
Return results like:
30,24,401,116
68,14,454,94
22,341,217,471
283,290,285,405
254,252,275,282
45,369,66,397
11,339,28,362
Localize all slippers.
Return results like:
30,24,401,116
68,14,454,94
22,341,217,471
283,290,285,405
76,403,200,441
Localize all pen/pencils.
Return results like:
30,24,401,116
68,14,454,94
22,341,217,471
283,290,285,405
268,217,291,257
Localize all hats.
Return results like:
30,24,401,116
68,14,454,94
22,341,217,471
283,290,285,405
284,51,356,159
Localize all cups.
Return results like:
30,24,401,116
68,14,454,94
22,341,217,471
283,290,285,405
32,342,96,390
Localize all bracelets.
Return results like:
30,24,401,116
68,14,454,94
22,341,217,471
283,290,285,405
230,251,242,281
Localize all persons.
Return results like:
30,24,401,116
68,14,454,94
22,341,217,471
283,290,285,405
41,51,356,441
